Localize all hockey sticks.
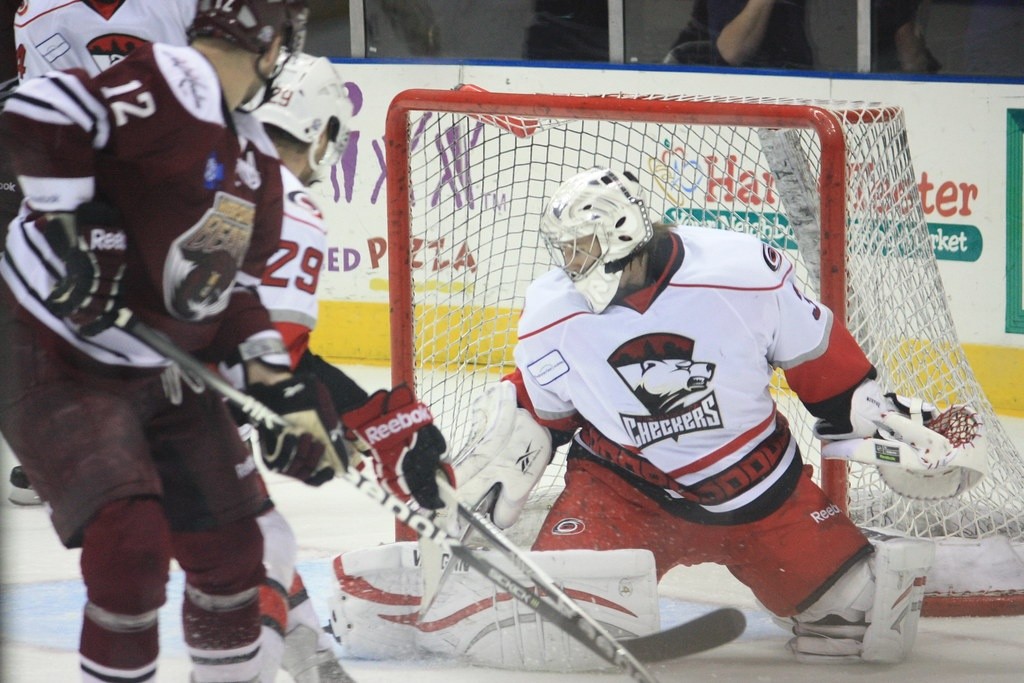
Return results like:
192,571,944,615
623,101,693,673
413,477,506,625
431,468,664,683
115,302,749,662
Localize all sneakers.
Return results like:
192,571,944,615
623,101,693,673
280,624,358,683
7,465,48,507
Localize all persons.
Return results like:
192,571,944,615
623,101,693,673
9,0,195,505
1,0,318,682
213,52,456,683
876,0,942,73
669,0,819,70
326,167,988,672
522,1,608,61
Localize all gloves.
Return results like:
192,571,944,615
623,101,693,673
340,381,450,511
240,372,353,487
33,197,145,339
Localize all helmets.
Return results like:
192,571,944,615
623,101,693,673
538,165,654,317
251,45,355,148
187,0,310,62
